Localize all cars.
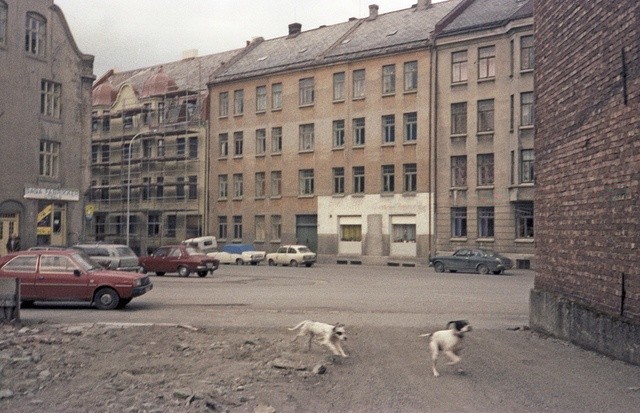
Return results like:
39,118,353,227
137,244,220,278
71,243,140,274
266,244,317,268
1,248,154,310
428,247,514,275
206,243,267,265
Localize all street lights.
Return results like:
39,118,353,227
125,128,157,247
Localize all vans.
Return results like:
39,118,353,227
183,235,217,253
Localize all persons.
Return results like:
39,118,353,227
6,233,20,253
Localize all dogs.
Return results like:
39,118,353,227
419,319,473,377
287,319,349,358
